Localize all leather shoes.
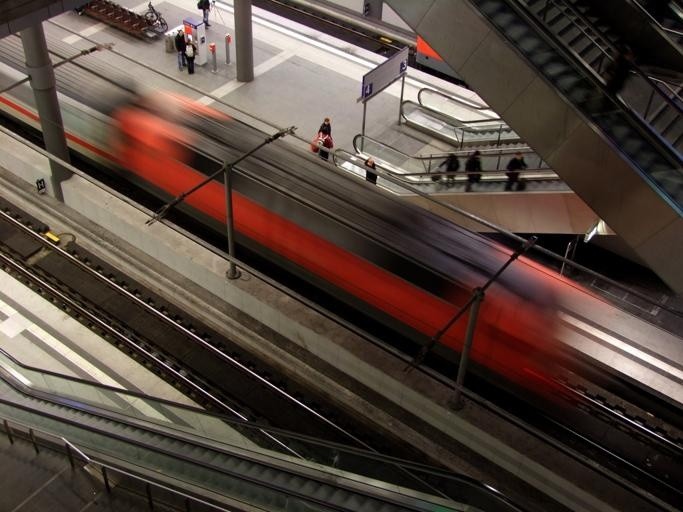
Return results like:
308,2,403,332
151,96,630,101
204,22,210,29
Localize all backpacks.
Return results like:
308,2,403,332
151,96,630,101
318,131,329,147
188,46,198,57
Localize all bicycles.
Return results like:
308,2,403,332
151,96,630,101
145,1,168,31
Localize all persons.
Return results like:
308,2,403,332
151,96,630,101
505,152,527,192
174,30,186,72
317,127,333,160
200,1,211,29
318,118,331,136
600,49,631,99
364,157,377,185
183,39,196,74
438,153,460,188
464,151,481,193
